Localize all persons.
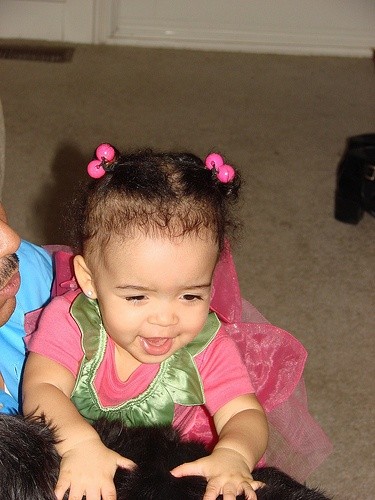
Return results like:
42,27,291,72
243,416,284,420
0,204,56,409
22,142,271,500
